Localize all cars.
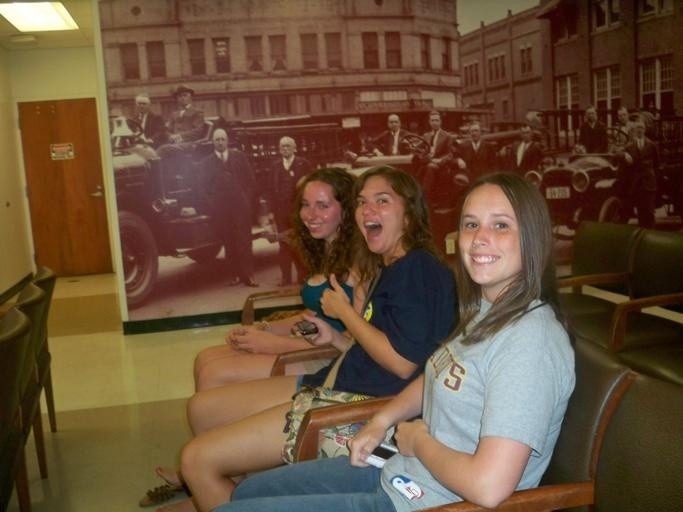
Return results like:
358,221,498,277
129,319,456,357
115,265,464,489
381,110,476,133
344,107,683,237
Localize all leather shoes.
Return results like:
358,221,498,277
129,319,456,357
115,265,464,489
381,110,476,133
231,277,259,286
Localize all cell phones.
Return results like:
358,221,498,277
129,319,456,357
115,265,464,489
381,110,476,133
371,444,398,462
296,320,318,335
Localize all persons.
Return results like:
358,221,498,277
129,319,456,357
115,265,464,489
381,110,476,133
132,83,663,288
209,171,576,512
156,167,383,488
155,165,460,512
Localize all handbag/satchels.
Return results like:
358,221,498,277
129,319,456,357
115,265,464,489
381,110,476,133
281,385,394,464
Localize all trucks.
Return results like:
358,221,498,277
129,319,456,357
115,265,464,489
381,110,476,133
109,110,342,309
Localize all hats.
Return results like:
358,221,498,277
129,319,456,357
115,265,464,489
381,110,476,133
173,87,193,99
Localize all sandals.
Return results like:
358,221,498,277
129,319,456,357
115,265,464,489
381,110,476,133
139,484,178,507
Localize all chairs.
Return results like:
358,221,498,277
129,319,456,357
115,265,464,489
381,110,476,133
0,266,65,512
240,215,681,512
